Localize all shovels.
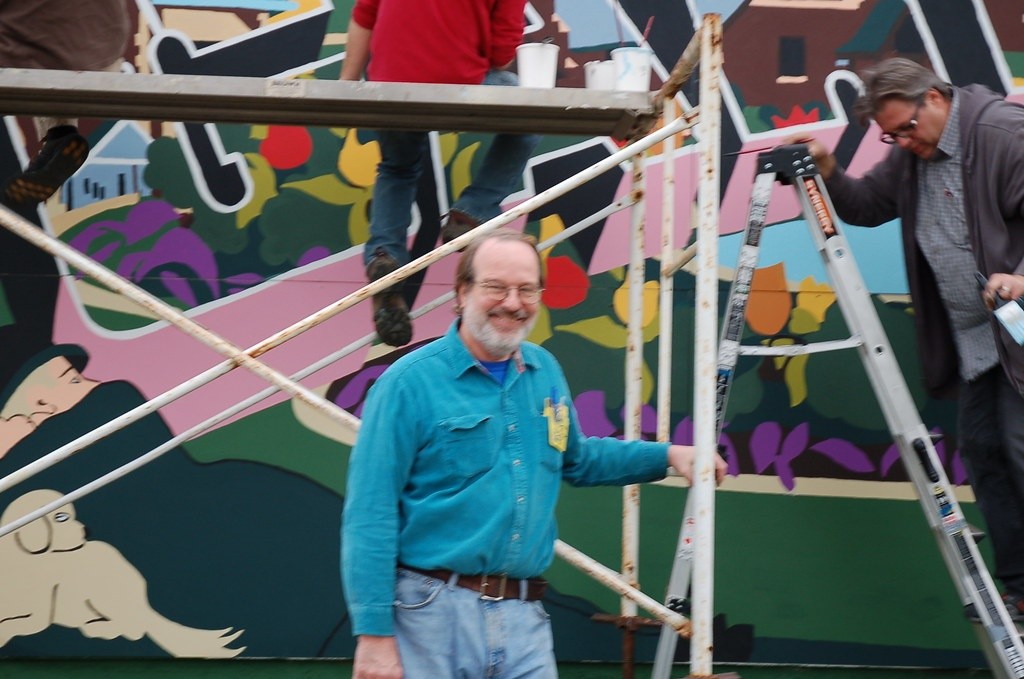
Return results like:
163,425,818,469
969,267,1024,348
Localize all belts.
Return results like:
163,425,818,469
397,560,549,602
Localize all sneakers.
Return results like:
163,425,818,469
1,129,87,205
366,255,413,347
443,205,474,255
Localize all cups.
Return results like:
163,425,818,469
584,60,613,90
609,47,653,91
515,42,559,88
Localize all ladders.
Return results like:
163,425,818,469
638,145,1024,679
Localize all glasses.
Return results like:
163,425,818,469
879,98,924,145
462,279,545,306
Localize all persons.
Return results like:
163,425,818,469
1,0,133,214
785,57,1024,624
336,229,729,679
337,0,530,348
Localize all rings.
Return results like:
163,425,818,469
1000,286,1012,293
